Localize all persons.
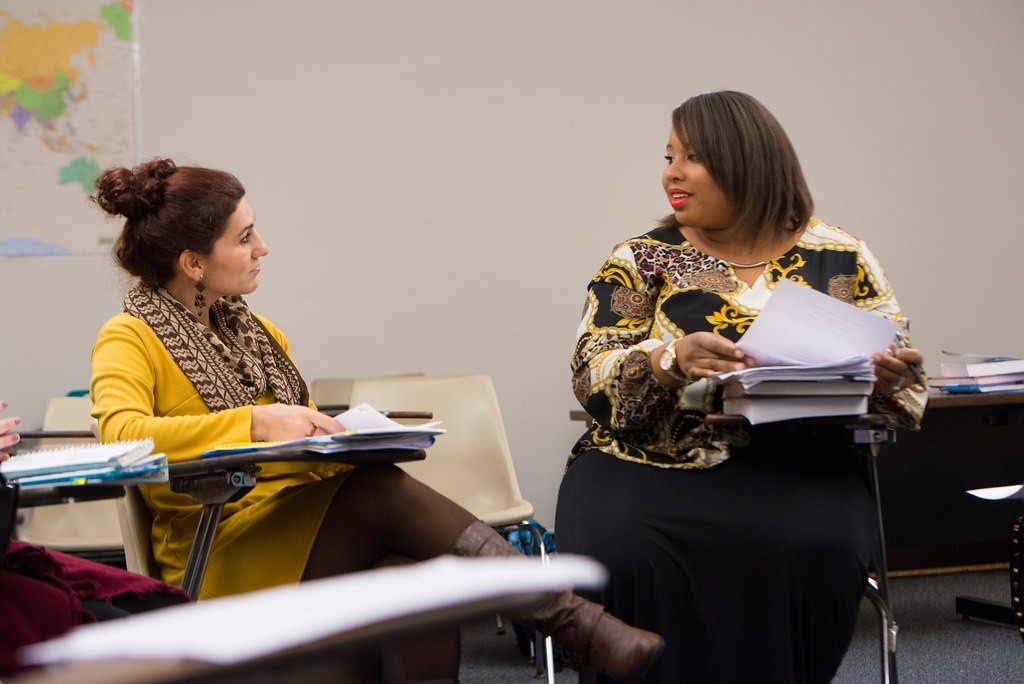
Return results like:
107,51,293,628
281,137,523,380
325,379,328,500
90,156,666,684
0,398,193,684
553,90,927,684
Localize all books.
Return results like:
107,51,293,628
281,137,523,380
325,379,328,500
1,440,168,489
927,350,1024,394
722,379,874,425
202,402,446,458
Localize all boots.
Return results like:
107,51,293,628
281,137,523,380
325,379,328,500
449,519,666,684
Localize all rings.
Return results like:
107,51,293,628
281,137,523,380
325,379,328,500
307,425,317,436
893,377,906,391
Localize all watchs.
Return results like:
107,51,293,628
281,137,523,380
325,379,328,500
660,338,689,382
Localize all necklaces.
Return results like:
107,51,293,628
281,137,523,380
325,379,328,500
727,261,768,268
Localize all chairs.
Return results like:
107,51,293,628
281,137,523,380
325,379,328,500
19,393,125,554
311,372,557,683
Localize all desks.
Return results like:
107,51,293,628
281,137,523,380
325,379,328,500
7,445,427,604
707,390,1024,684
